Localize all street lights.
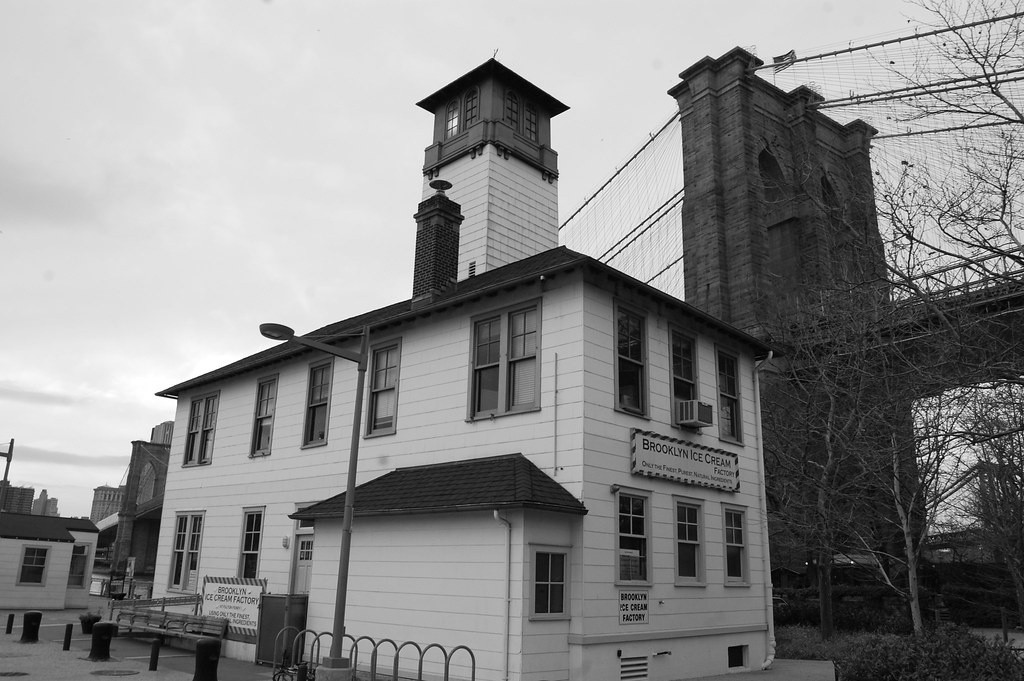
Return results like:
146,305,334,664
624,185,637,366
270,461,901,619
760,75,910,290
261,324,370,680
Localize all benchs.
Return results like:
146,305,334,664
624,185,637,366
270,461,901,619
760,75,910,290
114,608,228,641
111,592,127,600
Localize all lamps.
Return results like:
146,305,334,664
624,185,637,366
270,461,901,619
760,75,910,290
283,536,290,550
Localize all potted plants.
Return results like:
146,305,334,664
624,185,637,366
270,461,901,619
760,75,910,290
79,612,102,634
